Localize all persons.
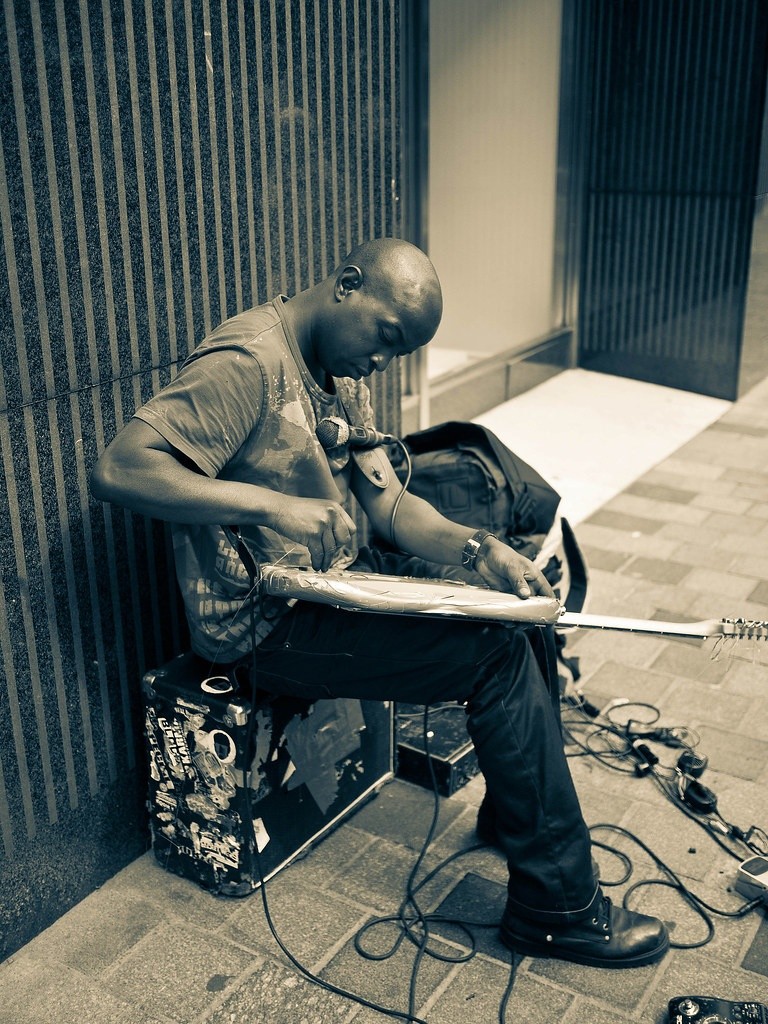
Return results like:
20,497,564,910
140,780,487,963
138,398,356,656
92,238,671,972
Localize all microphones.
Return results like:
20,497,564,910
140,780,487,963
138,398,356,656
317,416,397,449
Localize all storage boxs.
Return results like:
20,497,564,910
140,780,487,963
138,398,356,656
143,647,398,900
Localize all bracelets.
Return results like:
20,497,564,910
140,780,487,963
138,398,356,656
462,529,499,573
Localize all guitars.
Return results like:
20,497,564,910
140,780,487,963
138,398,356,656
260,566,768,662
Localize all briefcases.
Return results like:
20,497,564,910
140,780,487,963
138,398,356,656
142,651,397,894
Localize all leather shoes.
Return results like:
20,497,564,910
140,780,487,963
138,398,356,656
473,815,602,880
500,894,670,969
